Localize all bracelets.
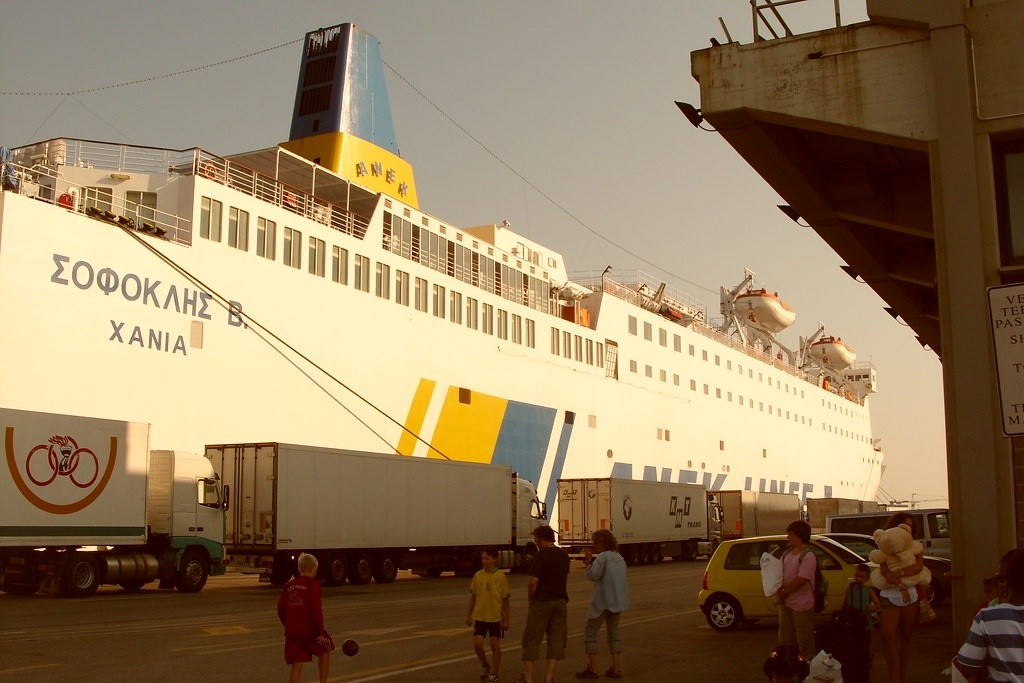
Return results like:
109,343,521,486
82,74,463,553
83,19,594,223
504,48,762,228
899,583,908,591
899,569,904,578
782,588,785,593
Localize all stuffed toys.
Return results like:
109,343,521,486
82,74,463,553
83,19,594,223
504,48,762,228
870,523,931,590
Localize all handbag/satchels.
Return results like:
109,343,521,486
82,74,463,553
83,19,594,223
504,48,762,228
760,551,784,595
917,582,937,624
803,649,844,683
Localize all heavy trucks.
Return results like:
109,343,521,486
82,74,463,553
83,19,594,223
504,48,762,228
705,489,809,543
203,442,547,586
556,477,725,567
0,407,229,600
806,497,888,535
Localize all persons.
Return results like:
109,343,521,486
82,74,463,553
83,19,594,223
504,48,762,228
515,525,570,683
577,529,628,678
465,545,510,683
762,512,1024,683
277,553,336,683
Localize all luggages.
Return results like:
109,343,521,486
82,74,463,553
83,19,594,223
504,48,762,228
831,582,873,683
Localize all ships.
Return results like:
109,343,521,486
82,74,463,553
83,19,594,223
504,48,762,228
0,11,886,549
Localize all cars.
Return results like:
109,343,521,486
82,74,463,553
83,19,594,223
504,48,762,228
698,535,881,639
817,532,951,602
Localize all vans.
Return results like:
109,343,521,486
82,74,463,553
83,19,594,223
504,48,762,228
825,508,950,561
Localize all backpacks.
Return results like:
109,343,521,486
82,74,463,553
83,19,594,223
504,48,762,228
783,547,826,613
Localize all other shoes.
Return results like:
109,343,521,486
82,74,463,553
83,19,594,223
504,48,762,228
576,664,600,678
480,663,491,679
544,674,554,683
488,673,499,683
516,674,528,683
605,667,621,678
